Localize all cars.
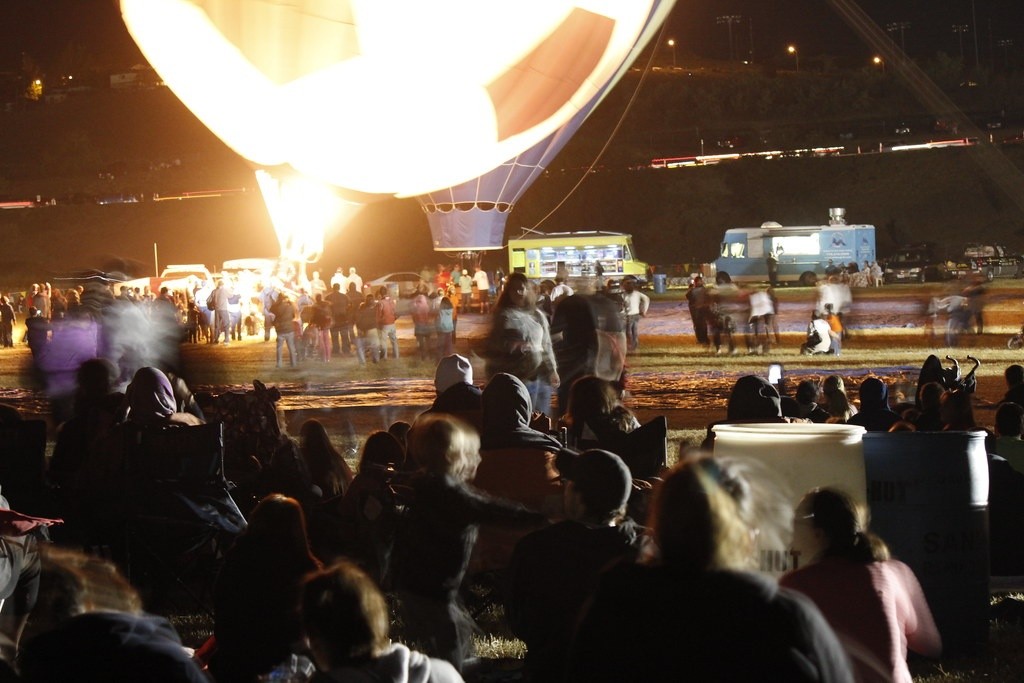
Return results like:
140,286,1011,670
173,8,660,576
160,264,216,292
944,240,1024,281
223,258,276,278
364,271,434,300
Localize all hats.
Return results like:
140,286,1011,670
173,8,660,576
553,446,633,514
462,268,469,276
434,352,475,394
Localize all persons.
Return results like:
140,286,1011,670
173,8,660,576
0,253,1024,683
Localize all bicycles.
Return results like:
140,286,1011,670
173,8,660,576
1007,321,1024,350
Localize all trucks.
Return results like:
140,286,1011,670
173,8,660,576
709,207,876,286
508,233,652,287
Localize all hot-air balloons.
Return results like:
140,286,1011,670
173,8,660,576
122,0,677,321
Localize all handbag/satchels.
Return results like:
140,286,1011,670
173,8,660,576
806,321,822,348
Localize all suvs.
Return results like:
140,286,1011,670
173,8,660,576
884,246,941,283
